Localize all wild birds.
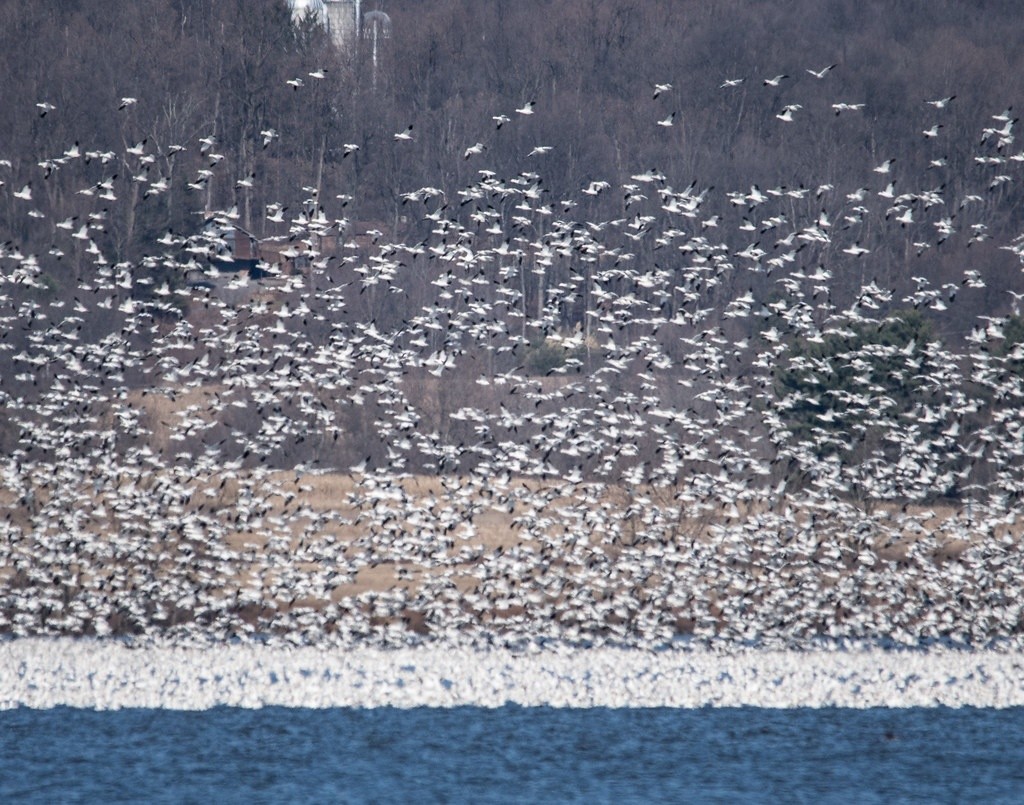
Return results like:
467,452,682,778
832,102,865,116
118,97,139,111
652,84,673,100
516,101,537,115
763,75,790,87
35,102,57,118
286,78,304,91
720,78,746,88
921,95,958,109
991,105,1013,121
776,104,803,121
309,69,329,80
0,112,1024,710
805,64,839,78
